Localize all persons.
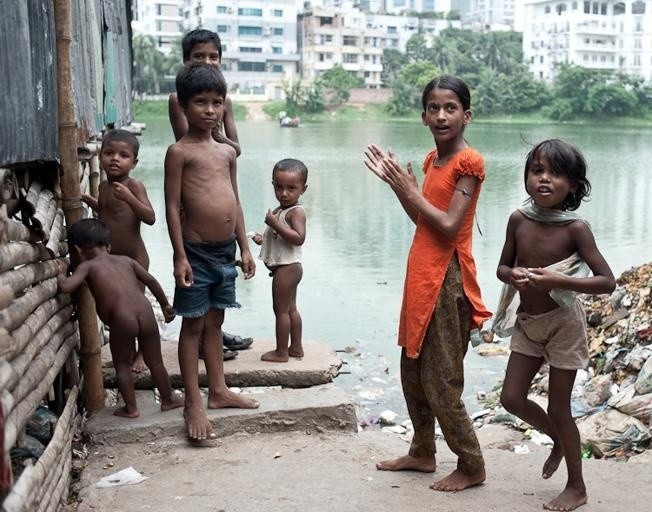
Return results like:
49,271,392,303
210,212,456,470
496,139,617,512
363,75,491,491
252,159,308,362
168,29,254,359
79,129,155,372
57,219,185,418
163,61,260,439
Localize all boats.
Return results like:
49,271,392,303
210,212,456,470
278,111,300,128
